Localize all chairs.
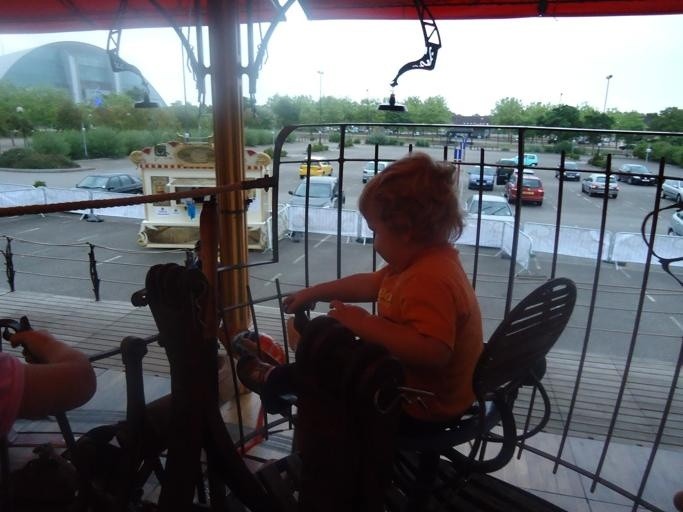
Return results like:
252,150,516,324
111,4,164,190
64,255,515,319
395,278,576,512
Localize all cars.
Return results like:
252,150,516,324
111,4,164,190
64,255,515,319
72,171,143,194
317,123,447,137
542,135,637,150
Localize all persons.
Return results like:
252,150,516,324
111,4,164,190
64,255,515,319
0,330,97,436
236,153,482,432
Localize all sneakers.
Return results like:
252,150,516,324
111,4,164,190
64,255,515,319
236,354,279,415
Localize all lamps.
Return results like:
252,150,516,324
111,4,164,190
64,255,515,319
134,84,160,108
376,83,408,113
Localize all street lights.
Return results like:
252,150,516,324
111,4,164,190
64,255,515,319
316,69,324,123
80,126,88,158
366,88,372,138
602,74,614,111
15,104,27,148
